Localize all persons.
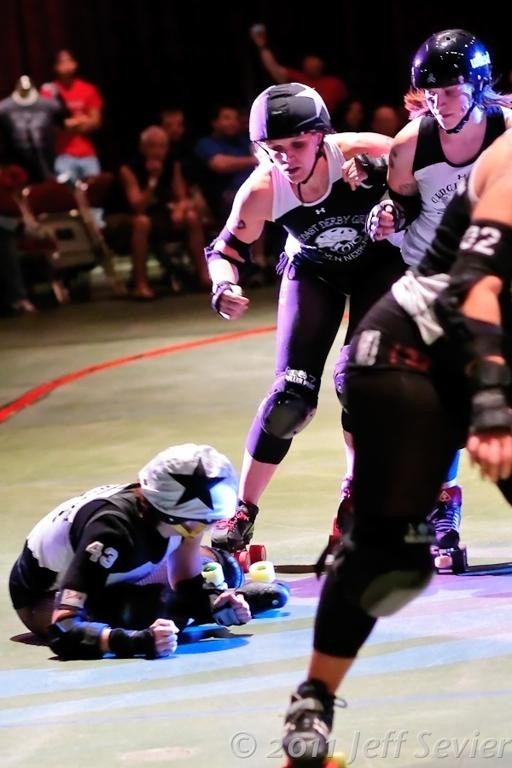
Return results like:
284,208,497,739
7,441,292,658
1,24,410,319
327,27,512,542
279,122,512,762
199,82,463,552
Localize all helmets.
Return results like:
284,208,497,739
411,28,493,93
248,82,332,142
138,443,240,520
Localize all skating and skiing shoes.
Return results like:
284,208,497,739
430,485,468,574
210,498,266,573
283,678,346,768
202,561,290,617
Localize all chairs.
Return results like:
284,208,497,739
12,174,189,306
